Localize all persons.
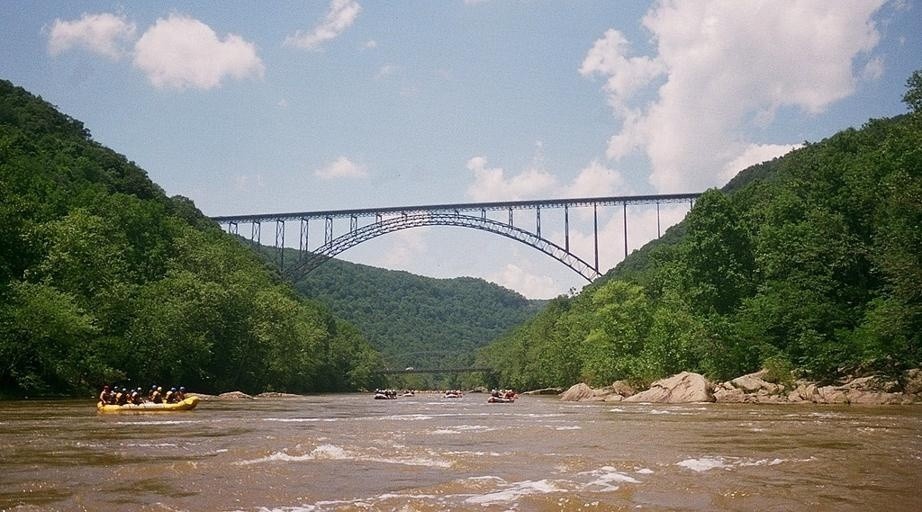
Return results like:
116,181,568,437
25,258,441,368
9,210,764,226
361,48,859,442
99,382,185,405
373,388,519,402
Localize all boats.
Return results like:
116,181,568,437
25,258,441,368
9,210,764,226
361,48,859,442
444,393,462,398
96,395,201,412
375,393,398,401
403,393,416,397
487,397,515,403
376,388,395,394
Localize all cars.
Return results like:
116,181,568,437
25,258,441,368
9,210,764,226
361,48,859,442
405,366,414,370
